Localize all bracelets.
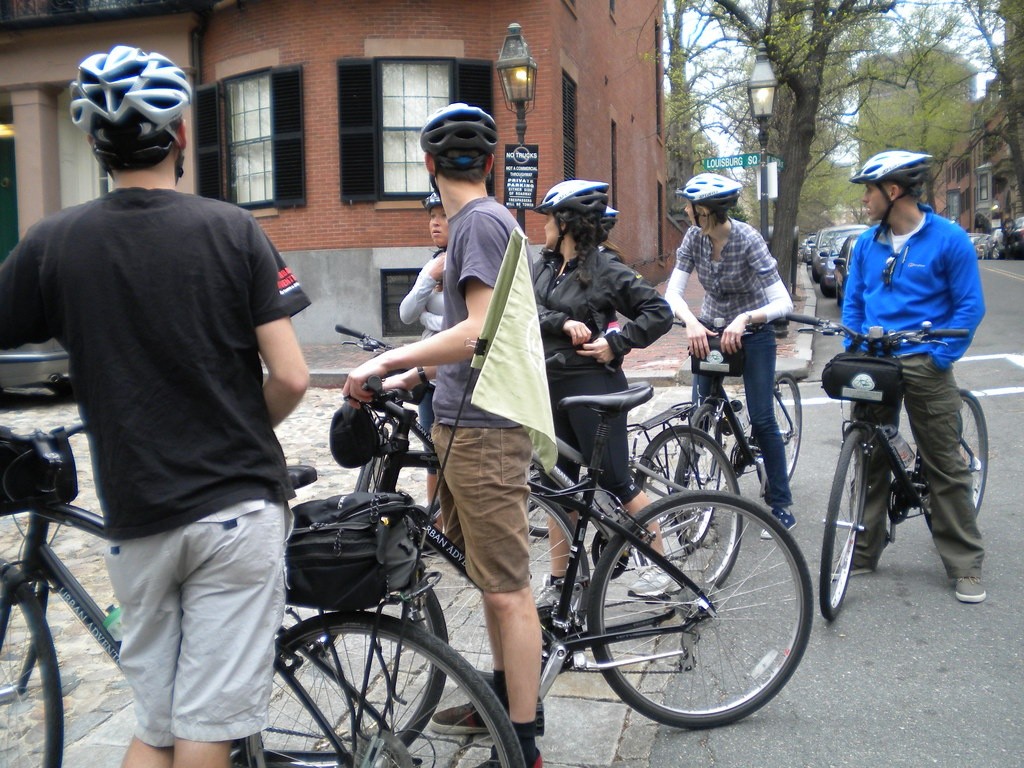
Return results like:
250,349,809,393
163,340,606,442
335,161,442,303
416,367,429,386
742,311,752,327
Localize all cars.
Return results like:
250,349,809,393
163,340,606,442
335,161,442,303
0,337,73,401
968,215,1024,258
798,225,872,308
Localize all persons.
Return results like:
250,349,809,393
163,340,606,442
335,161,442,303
664,173,796,540
341,101,548,768
0,47,308,768
530,179,681,610
819,150,986,602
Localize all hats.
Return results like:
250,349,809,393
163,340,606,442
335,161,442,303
329,399,380,468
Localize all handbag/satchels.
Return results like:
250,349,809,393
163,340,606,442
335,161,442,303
285,489,430,611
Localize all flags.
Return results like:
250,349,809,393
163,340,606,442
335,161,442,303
468,228,559,475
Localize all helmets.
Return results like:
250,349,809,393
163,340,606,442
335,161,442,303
422,192,442,210
420,103,498,156
533,180,610,218
849,151,933,189
70,45,191,144
675,173,742,211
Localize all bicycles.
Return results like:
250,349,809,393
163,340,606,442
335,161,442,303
785,312,989,623
0,411,538,768
669,307,803,554
240,321,814,762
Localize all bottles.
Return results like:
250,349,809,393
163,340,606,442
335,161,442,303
883,423,915,467
580,476,619,523
730,400,749,432
103,604,123,648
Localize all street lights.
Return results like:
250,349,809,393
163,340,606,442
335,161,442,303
746,36,780,248
496,22,549,234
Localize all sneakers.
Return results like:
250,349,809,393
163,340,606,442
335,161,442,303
955,577,986,602
428,701,488,735
475,745,543,768
760,508,797,540
628,564,682,598
535,574,580,609
818,559,872,580
